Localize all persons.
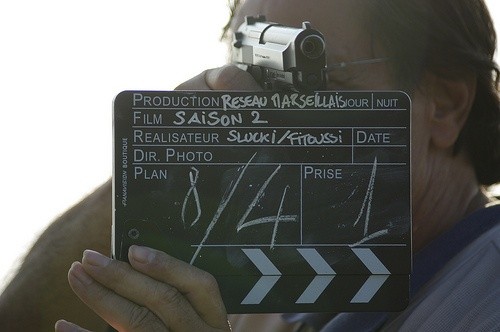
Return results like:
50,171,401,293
0,0,500,330
